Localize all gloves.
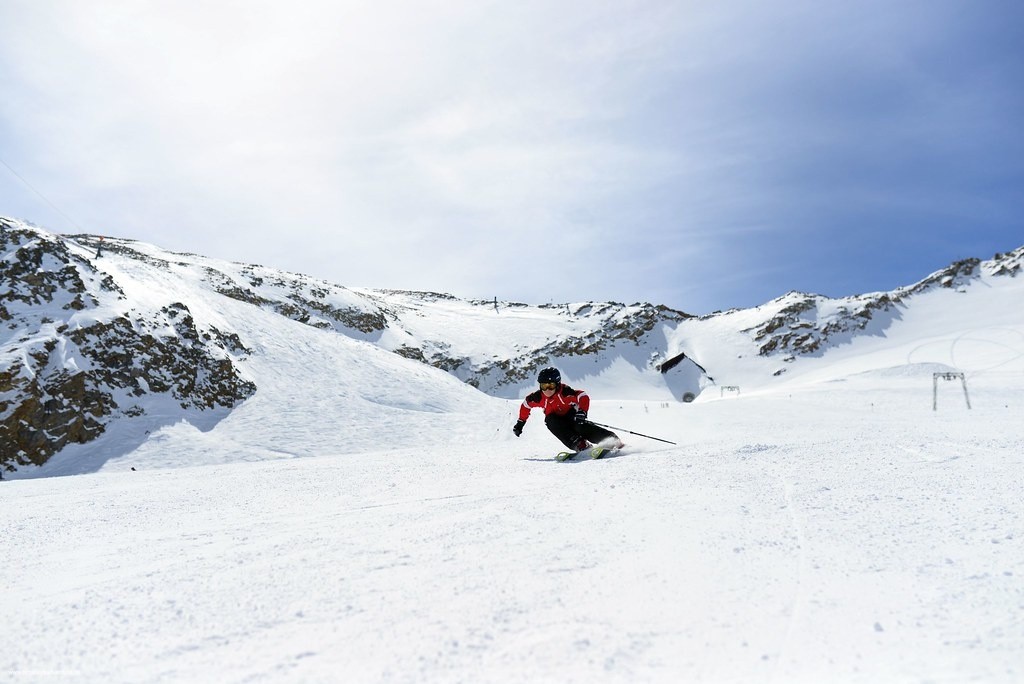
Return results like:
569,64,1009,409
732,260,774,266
575,409,587,427
513,418,526,437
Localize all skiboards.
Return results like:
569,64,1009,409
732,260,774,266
555,444,626,462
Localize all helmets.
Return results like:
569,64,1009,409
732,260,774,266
538,367,561,385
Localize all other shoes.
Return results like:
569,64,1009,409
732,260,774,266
572,437,623,459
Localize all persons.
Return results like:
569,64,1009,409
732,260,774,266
513,367,620,453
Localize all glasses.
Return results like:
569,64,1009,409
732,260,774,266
541,385,556,391
